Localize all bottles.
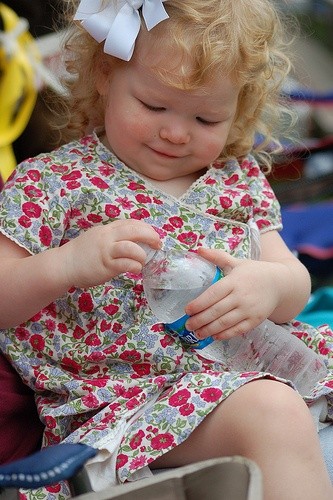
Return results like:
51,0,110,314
134,241,328,398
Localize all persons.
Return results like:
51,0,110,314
1,0,333,500
